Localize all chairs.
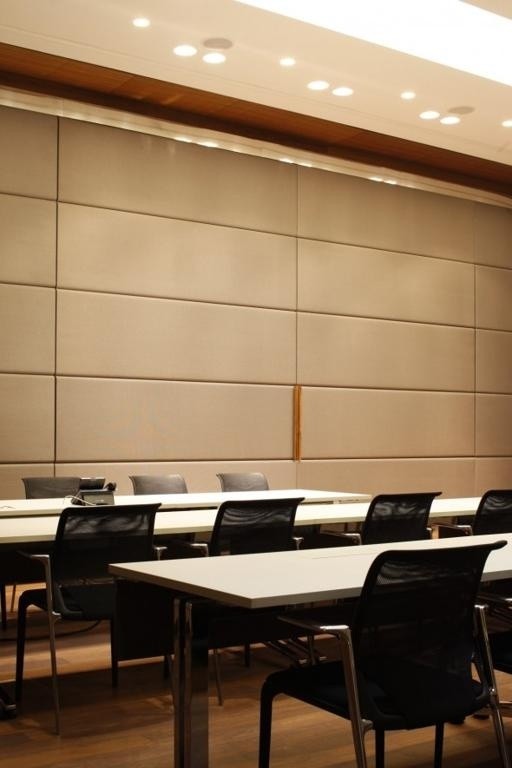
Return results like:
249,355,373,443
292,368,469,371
439,489,512,537
128,473,195,543
484,586,512,622
216,472,269,491
162,497,317,705
15,502,174,735
319,491,442,547
10,476,80,612
259,539,510,767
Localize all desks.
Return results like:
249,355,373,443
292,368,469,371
0,488,512,625
107,532,512,767
